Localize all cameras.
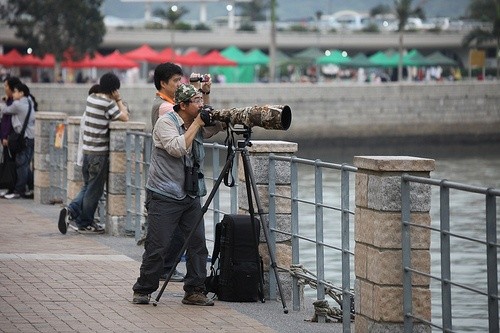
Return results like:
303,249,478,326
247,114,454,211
197,104,292,131
189,73,210,82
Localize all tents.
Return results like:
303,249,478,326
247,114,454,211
0,45,459,82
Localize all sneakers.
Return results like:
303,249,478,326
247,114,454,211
58,207,72,235
181,290,215,306
77,221,105,234
132,291,152,304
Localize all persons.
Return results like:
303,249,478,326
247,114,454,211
132,84,227,307
0,62,243,280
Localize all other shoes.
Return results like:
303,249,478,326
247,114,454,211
0,190,20,199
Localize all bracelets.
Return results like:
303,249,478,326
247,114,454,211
201,89,210,95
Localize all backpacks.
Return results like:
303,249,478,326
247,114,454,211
209,213,262,276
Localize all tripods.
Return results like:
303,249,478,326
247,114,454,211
153,127,288,313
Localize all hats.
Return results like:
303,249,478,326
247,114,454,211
173,83,201,106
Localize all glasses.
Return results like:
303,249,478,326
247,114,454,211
188,99,203,104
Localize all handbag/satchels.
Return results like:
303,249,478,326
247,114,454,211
206,273,260,303
7,129,27,156
